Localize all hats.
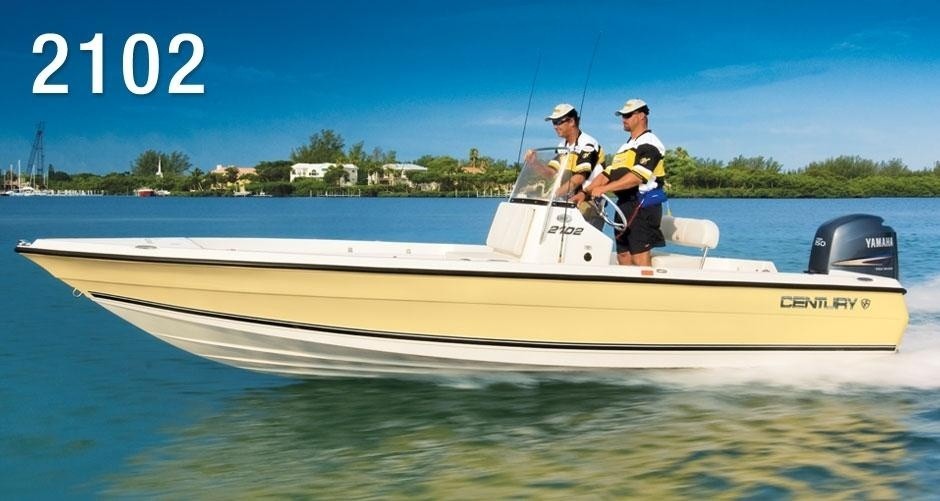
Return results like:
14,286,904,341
543,104,576,122
615,99,647,117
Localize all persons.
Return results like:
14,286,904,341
523,103,608,233
568,98,665,265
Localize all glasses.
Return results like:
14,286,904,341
622,113,633,119
552,119,570,126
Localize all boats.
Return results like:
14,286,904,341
13,54,910,391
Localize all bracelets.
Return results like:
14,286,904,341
580,189,587,201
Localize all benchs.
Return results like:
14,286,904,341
610,216,720,269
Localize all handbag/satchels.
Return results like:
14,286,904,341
635,186,669,209
